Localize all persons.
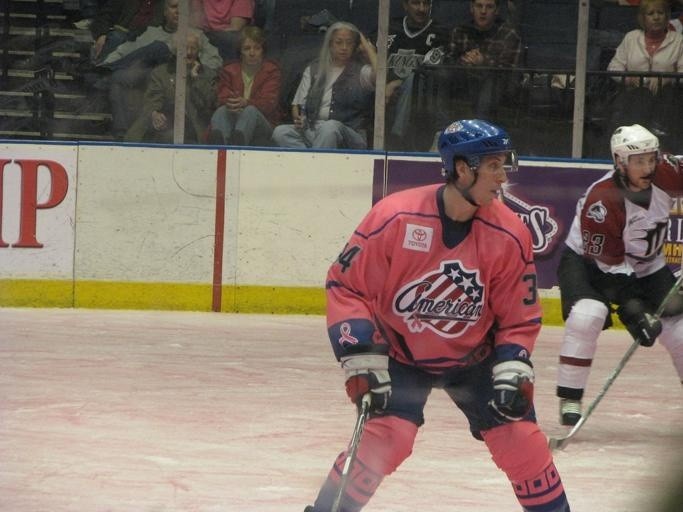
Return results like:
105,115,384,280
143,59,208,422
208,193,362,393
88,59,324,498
553,121,683,430
88,0,682,162
299,116,571,512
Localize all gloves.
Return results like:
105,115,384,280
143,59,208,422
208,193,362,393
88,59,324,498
339,342,393,420
482,356,545,427
616,298,662,346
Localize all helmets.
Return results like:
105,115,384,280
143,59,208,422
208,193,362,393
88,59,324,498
610,123,660,167
438,119,519,180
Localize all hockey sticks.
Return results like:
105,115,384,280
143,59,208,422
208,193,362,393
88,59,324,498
547,276,683,449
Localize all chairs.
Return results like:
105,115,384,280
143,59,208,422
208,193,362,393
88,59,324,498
511,2,651,143
